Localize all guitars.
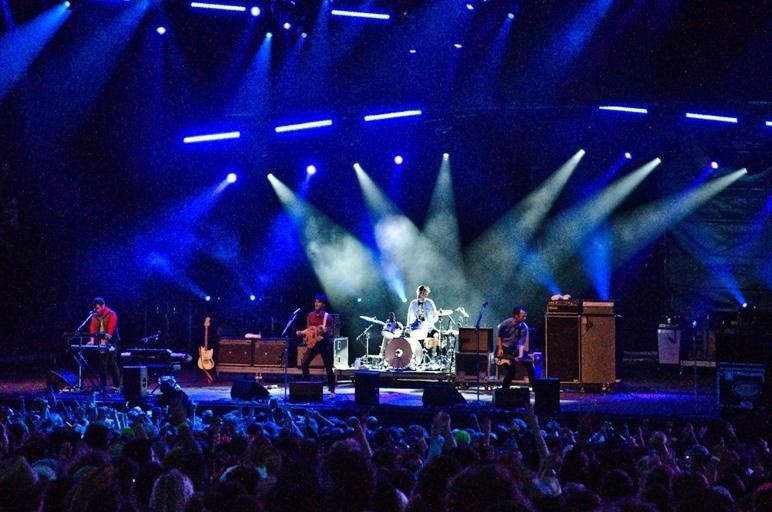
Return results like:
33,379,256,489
494,351,540,366
306,322,343,348
198,317,215,370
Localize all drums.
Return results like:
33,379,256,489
441,330,459,351
425,337,441,348
384,337,423,370
409,320,428,340
381,321,403,340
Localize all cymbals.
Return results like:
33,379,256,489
360,316,385,325
430,310,453,316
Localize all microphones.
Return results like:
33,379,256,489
89,308,96,314
483,302,488,308
293,308,301,314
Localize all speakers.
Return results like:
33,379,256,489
422,384,467,408
216,336,252,367
230,380,269,401
546,314,616,386
458,327,494,352
492,387,530,411
455,352,491,380
252,338,296,368
355,372,380,407
41,360,79,391
289,382,323,403
534,377,560,417
123,366,147,400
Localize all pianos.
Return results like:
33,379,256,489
64,332,112,339
69,345,116,351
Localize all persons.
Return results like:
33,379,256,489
1,380,772,511
404,284,454,364
495,305,538,392
295,294,337,400
386,311,404,331
85,297,122,390
